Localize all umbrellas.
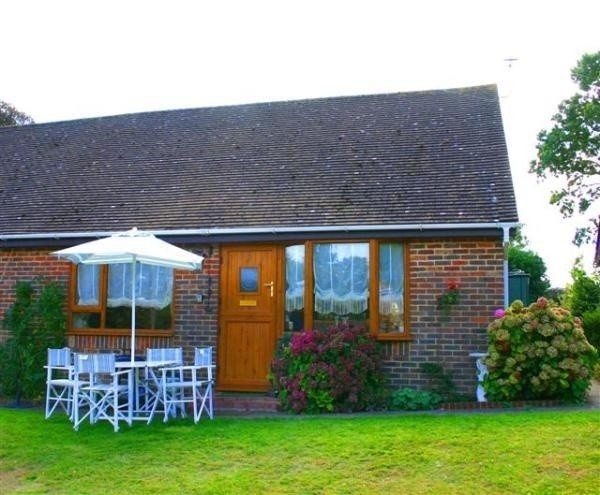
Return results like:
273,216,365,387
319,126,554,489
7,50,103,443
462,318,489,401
47,225,205,362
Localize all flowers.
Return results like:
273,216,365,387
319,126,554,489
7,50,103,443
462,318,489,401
438,279,460,306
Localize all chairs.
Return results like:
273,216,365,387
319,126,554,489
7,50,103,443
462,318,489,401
42,345,218,433
